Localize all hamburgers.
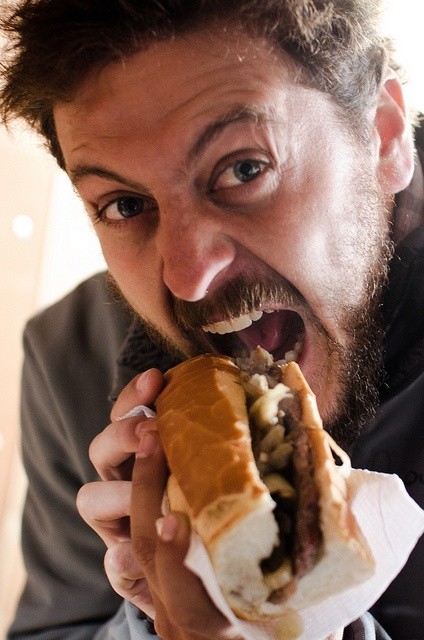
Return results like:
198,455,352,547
156,347,377,623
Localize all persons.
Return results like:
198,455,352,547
0,1,424,640
